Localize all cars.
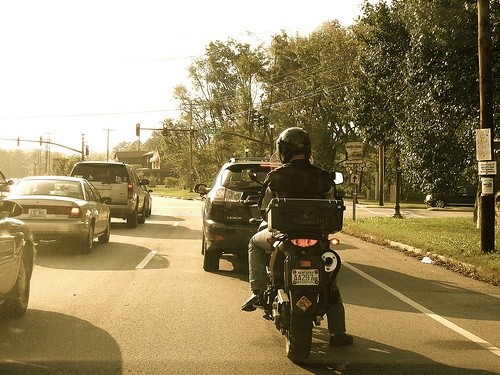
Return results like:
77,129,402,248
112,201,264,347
424,186,475,208
4,175,112,255
0,170,35,319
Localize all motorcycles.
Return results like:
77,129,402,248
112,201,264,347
240,167,347,366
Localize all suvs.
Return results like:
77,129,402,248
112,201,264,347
70,160,154,228
195,158,283,273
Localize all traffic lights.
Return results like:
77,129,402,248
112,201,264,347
136,123,140,136
190,125,194,138
163,124,167,136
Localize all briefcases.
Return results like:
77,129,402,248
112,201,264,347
266,198,343,232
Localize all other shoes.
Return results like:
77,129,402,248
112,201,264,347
330,333,353,345
241,293,266,311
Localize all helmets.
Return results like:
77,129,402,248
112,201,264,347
276,127,311,163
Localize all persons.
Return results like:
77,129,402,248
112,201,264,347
241,127,353,348
